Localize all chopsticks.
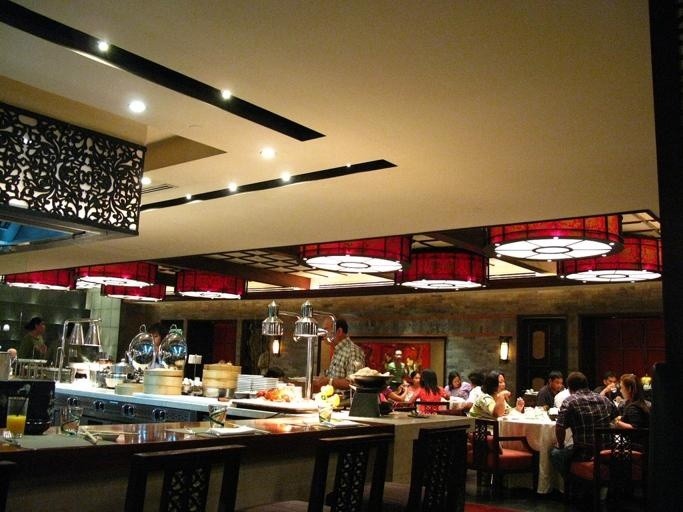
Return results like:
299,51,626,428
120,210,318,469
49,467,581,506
80,426,97,443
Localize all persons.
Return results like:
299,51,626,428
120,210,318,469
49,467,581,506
18,317,47,359
7,348,17,367
149,322,167,346
380,348,652,482
264,366,285,379
311,317,366,506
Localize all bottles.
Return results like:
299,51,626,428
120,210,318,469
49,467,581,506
193,377,202,393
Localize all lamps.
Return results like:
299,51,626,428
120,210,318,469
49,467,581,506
3,258,246,305
271,333,282,358
557,235,661,284
394,247,489,290
49,317,102,384
487,214,624,262
496,334,512,364
260,298,340,398
297,235,412,273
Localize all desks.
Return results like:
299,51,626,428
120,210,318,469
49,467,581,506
490,407,565,492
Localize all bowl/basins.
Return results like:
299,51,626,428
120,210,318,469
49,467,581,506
88,357,130,389
379,401,393,415
24,416,51,434
346,374,393,392
248,393,255,399
233,392,249,398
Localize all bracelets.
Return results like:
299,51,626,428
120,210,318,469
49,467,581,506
329,377,334,385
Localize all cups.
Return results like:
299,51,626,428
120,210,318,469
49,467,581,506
205,386,219,397
317,402,333,423
0,352,11,381
5,397,28,438
610,385,617,392
59,406,82,439
208,405,226,430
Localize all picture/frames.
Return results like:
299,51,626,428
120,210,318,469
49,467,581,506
314,333,448,393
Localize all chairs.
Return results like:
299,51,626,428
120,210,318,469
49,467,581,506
466,418,540,508
240,430,396,511
117,442,248,511
562,426,650,504
249,424,468,512
0,458,16,511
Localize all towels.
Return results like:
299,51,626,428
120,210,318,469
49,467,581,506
206,425,255,436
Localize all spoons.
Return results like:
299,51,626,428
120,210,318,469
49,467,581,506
183,425,220,437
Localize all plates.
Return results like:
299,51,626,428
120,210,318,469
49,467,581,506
408,413,430,417
85,431,121,440
236,374,277,393
209,424,256,436
